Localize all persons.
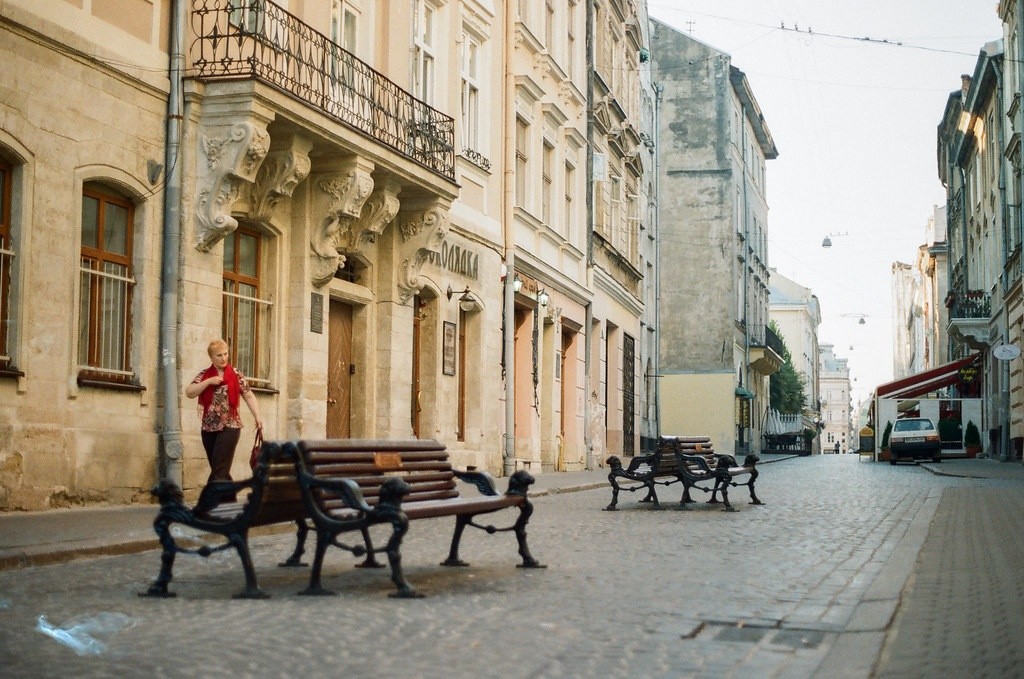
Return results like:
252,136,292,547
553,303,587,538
835,441,840,454
185,339,263,502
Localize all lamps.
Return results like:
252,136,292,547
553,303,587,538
858,317,866,325
446,285,477,312
513,272,523,295
821,235,833,248
849,346,854,351
538,287,550,305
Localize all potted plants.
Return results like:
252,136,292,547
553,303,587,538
963,420,981,457
880,420,891,460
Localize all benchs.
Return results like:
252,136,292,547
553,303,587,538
139,441,386,600
283,436,547,600
601,435,697,512
674,436,766,513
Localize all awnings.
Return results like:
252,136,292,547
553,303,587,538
867,350,983,461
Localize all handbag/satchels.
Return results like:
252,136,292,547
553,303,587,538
250,428,264,471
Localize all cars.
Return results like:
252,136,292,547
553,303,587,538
888,418,942,465
